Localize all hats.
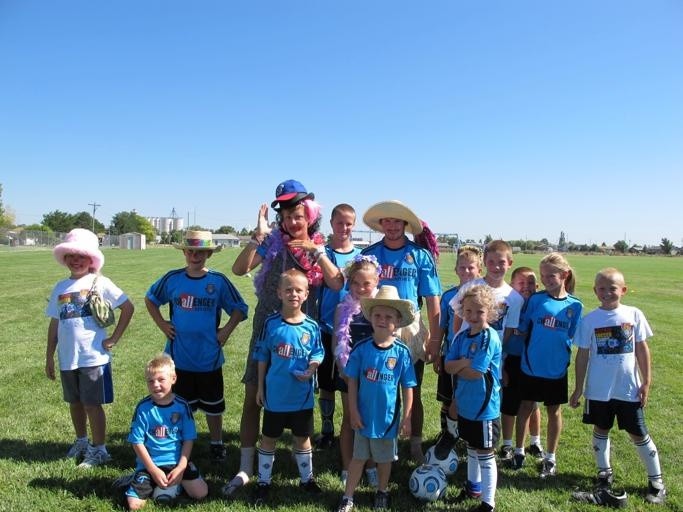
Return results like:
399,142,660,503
359,284,416,328
361,199,424,236
271,179,315,213
54,228,105,273
171,229,224,254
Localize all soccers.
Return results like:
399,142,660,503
424,445,459,476
409,464,447,501
153,483,182,504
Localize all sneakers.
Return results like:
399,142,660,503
595,472,614,491
110,475,131,492
298,478,322,496
336,466,392,512
500,442,557,481
314,434,335,455
645,479,666,506
434,426,461,460
409,454,426,464
209,441,227,462
252,475,272,506
67,437,112,470
442,483,481,506
468,501,493,512
570,486,629,510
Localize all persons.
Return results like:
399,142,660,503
44,227,134,469
143,229,248,462
112,357,209,510
570,268,667,503
220,180,584,512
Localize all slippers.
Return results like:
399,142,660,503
222,481,240,497
